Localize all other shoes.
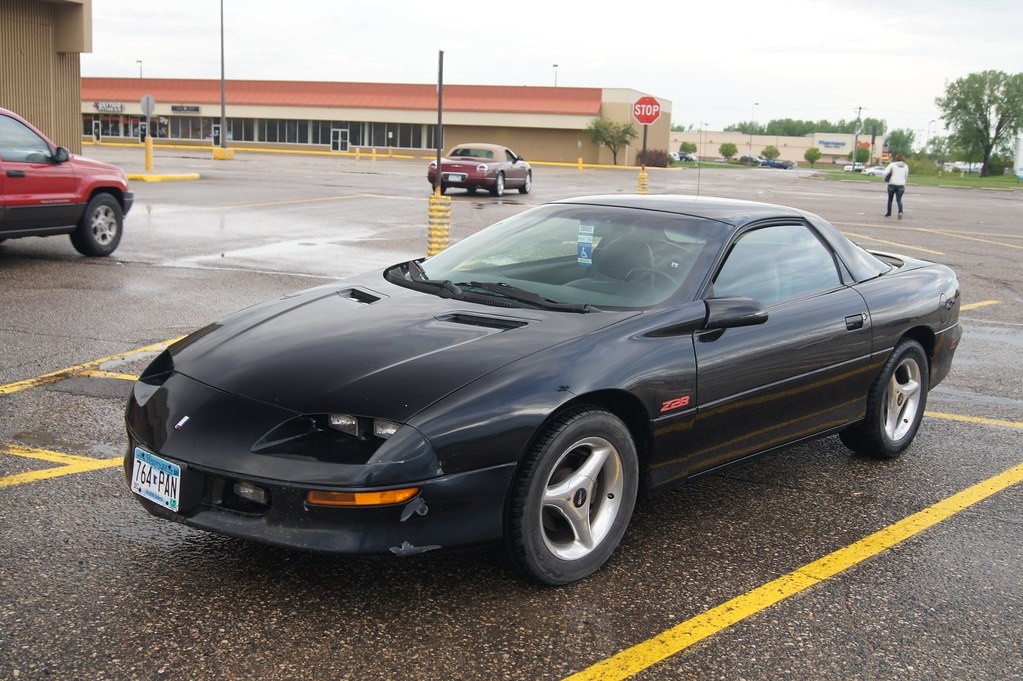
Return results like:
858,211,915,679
898,213,902,219
885,213,893,217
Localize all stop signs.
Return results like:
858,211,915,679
632,97,661,125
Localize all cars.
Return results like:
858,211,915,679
762,159,798,169
861,166,887,176
671,152,699,162
1,106,136,259
715,156,738,162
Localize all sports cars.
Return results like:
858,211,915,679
120,194,961,582
426,143,533,194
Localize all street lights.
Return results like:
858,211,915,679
703,123,709,159
553,65,558,86
749,103,759,165
927,120,936,156
136,60,142,79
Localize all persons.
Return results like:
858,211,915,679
883,154,909,219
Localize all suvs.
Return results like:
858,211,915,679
740,155,766,163
844,162,865,172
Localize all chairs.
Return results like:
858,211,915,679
715,253,781,306
487,151,493,158
460,149,472,155
590,236,655,287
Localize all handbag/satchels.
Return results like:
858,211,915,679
886,173,891,182
